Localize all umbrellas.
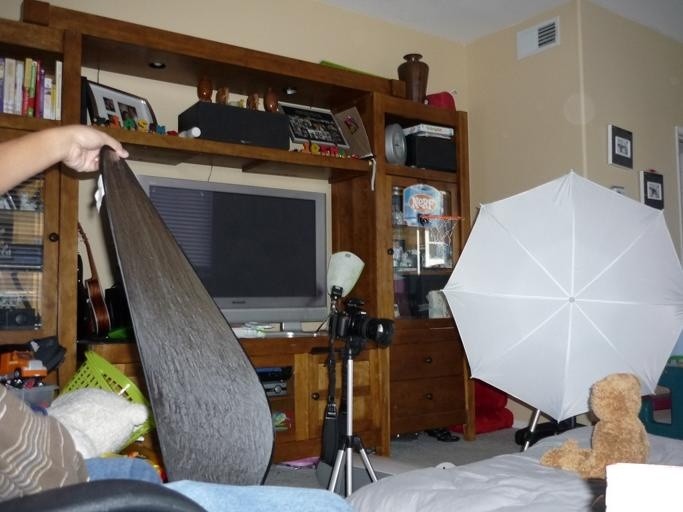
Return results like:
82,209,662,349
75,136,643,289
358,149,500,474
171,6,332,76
439,169,682,425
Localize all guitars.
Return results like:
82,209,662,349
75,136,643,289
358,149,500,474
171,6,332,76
78,222,111,335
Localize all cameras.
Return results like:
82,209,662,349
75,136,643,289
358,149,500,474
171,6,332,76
328,298,394,357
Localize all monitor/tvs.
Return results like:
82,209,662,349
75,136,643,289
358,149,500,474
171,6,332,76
136,173,329,336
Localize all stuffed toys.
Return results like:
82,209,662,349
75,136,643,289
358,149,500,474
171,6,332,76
539,372,650,481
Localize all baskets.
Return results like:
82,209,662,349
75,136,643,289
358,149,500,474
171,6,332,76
59,351,154,454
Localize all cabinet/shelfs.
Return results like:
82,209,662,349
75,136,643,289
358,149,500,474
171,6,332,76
0,1,475,464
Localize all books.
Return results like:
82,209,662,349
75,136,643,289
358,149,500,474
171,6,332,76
0,209,44,273
0,54,62,124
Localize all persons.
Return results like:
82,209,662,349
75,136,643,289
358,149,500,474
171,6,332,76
126,106,137,125
122,111,136,129
0,121,164,500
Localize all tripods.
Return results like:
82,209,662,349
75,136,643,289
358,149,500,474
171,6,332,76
323,362,376,499
